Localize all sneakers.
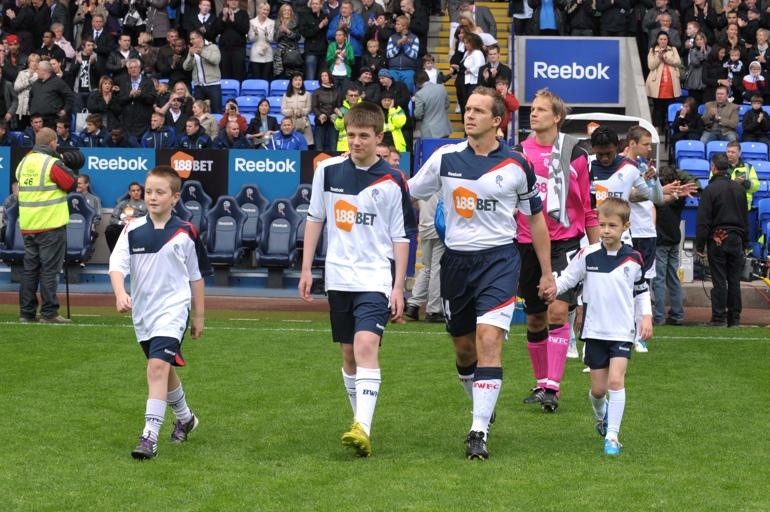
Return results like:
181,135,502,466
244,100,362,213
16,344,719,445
171,411,199,443
566,336,579,359
604,438,621,456
342,429,372,457
524,387,559,412
489,411,496,424
19,315,73,325
595,399,608,437
425,313,447,323
634,335,648,353
132,438,157,458
406,307,419,320
467,429,489,460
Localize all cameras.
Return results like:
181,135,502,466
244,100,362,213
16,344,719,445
230,104,234,111
178,97,184,102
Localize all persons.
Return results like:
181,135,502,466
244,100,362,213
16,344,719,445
106,165,210,461
409,86,558,462
0,0,770,373
296,100,413,460
535,194,653,458
518,87,602,416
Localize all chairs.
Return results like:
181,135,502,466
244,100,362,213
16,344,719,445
2,79,415,288
665,79,770,258
